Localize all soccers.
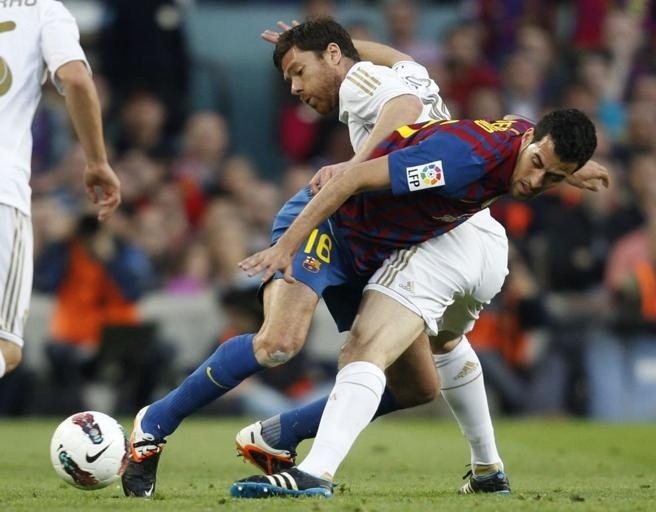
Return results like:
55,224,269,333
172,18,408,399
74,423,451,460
50,411,131,491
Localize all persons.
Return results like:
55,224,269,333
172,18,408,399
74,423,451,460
229,16,515,499
1,1,122,378
16,1,654,418
119,106,609,502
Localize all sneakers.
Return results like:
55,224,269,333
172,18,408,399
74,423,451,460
235,421,296,475
121,405,167,497
457,469,511,495
230,467,339,497
